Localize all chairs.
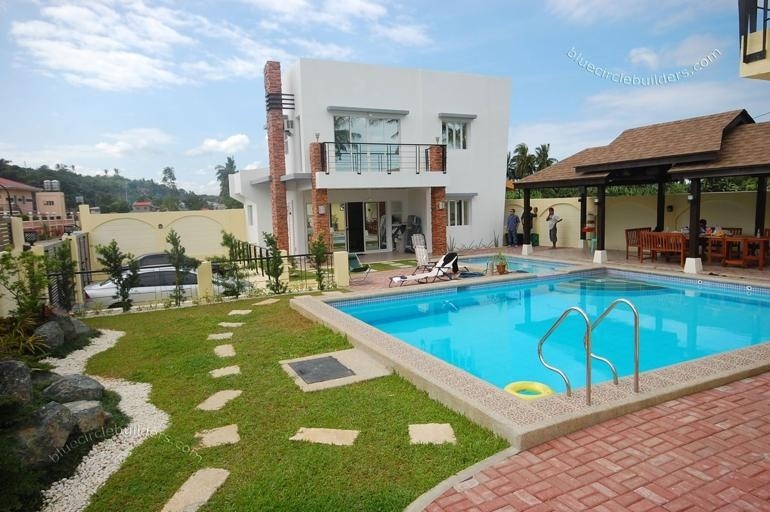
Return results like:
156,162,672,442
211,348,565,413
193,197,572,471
348,253,374,284
413,246,437,274
388,254,457,287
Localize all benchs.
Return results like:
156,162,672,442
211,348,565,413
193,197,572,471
624,227,743,268
764,228,770,237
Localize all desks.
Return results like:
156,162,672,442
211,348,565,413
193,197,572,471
742,237,770,270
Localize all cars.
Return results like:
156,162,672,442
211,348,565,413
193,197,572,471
118,252,231,276
83,264,238,308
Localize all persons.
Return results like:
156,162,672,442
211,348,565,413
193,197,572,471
504,209,520,248
520,206,538,244
545,207,563,248
699,218,707,256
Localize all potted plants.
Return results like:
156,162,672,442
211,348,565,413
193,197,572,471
493,249,510,274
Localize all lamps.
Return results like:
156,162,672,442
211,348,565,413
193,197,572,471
25,230,37,247
439,202,447,211
319,205,325,215
316,132,319,142
594,196,600,206
65,226,75,236
577,193,583,202
686,193,695,201
666,205,674,212
436,137,440,143
